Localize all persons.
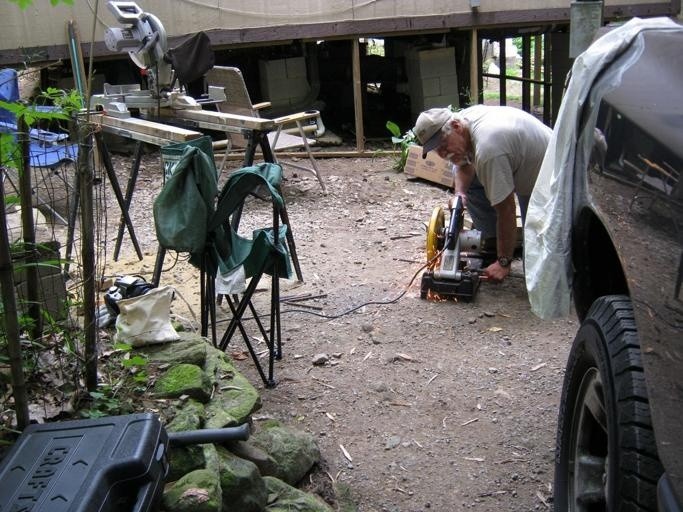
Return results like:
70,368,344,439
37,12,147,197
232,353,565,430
413,103,554,285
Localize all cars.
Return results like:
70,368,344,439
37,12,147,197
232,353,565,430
552,15,683,512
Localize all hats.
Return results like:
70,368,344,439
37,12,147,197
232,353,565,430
412,108,453,159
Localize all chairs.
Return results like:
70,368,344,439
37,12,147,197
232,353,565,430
204,64,329,197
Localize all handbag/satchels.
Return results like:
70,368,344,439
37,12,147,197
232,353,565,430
113,284,181,349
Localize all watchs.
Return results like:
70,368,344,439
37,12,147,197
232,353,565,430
496,255,514,267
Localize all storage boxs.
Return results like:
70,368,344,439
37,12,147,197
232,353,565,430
402,146,454,186
